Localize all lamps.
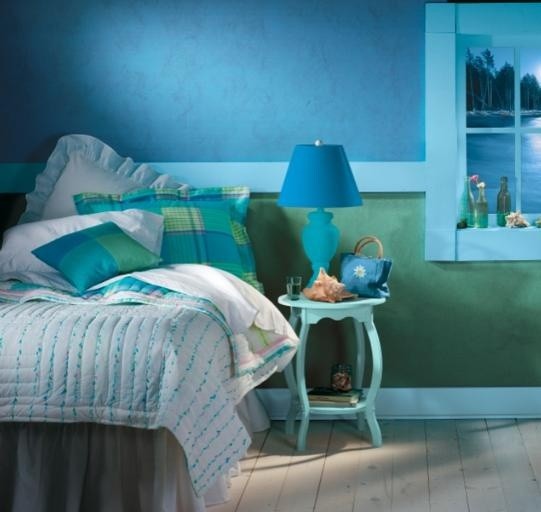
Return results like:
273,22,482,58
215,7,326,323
274,140,364,290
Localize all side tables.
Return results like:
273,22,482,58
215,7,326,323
277,292,386,453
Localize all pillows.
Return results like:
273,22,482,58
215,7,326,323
73,186,265,296
16,134,191,226
32,222,163,292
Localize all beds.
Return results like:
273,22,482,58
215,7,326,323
1,264,299,511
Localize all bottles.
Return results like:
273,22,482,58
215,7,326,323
496,175,512,228
474,185,488,229
459,175,475,226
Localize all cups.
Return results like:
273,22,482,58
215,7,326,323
283,274,301,300
329,360,353,392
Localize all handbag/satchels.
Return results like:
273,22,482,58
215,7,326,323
339,237,391,298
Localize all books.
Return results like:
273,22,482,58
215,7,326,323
307,386,363,408
